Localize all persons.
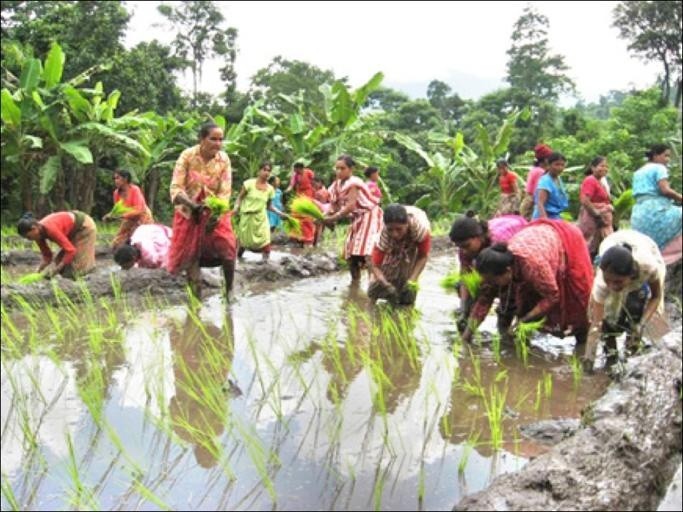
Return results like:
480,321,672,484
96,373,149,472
320,307,422,413
102,170,154,249
438,360,584,458
449,210,666,374
231,153,385,287
167,307,235,469
577,154,616,263
496,142,569,222
367,202,431,306
114,225,174,272
14,210,97,280
168,126,238,305
631,144,682,303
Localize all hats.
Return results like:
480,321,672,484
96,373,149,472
533,144,553,160
